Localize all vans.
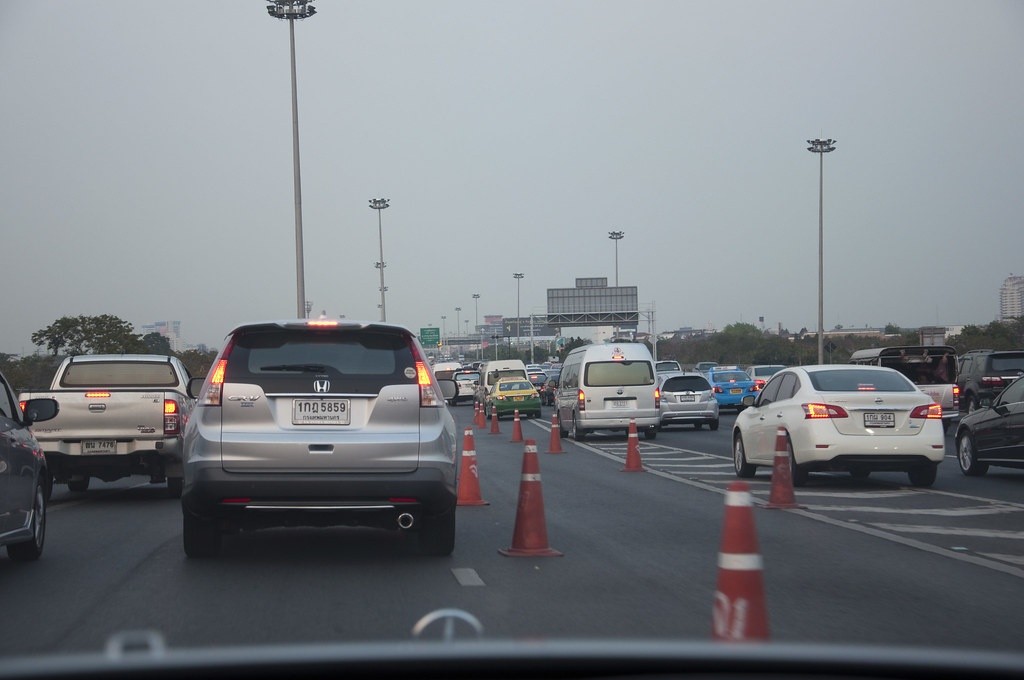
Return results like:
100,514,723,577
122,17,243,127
431,363,463,381
653,360,683,386
548,342,661,441
481,359,530,404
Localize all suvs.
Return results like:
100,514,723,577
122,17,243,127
958,349,1024,415
181,320,460,557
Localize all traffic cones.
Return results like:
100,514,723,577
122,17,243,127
477,402,488,429
619,417,648,473
456,425,490,507
761,425,808,510
472,401,480,425
709,481,772,644
543,413,567,455
498,440,565,558
509,409,527,444
488,406,503,435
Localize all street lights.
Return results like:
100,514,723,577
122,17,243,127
265,0,317,319
805,137,837,365
472,293,480,325
305,301,314,319
441,315,446,346
608,230,624,287
512,272,525,341
367,198,390,320
455,307,461,345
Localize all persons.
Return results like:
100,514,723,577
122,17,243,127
922,349,950,384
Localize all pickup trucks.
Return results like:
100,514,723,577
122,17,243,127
17,352,198,499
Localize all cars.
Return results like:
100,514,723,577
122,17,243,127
447,371,482,406
745,365,787,393
954,375,1024,476
730,363,947,490
0,372,62,565
424,345,846,406
485,377,542,419
656,371,719,433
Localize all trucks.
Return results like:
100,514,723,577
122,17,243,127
848,345,960,429
